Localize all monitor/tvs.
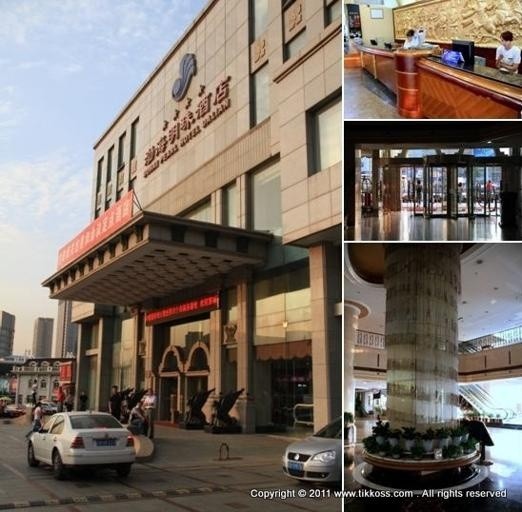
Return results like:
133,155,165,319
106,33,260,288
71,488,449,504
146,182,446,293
371,39,377,45
452,40,474,64
384,43,392,49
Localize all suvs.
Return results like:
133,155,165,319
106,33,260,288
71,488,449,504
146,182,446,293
40,401,58,414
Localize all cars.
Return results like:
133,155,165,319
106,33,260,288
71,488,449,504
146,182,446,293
402,181,494,206
24,407,136,481
279,415,342,488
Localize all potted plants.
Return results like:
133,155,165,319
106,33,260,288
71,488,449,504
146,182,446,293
360,414,482,459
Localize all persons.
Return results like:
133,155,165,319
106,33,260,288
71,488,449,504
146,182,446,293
494,31,521,75
456,181,464,206
31,401,43,430
414,179,423,206
485,179,496,203
402,29,415,49
31,379,38,405
54,386,66,412
79,389,88,412
107,384,159,439
63,390,74,413
